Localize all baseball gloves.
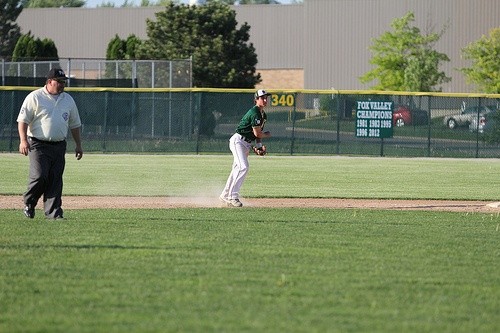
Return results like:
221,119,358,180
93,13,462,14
254,145,266,156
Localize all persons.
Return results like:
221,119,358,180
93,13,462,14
219,89,271,207
16,68,83,219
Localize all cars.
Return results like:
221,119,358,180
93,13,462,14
393,103,428,128
442,104,500,133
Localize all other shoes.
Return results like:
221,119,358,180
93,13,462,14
56,215,67,220
219,194,242,207
23,205,35,219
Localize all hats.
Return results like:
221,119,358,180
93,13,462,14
254,89,272,100
48,68,70,81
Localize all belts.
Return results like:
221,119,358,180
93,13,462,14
241,136,252,143
